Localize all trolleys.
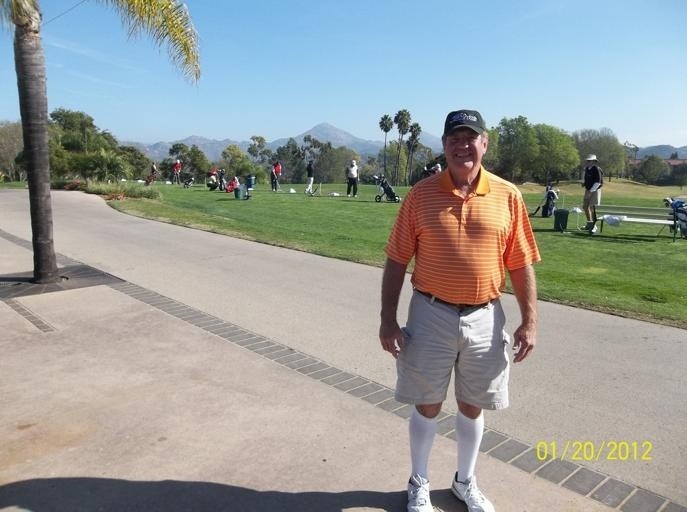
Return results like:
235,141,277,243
373,172,401,203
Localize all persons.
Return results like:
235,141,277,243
303,160,314,194
206,168,257,193
270,163,279,192
274,161,281,182
344,159,360,198
423,157,441,175
171,159,181,185
151,162,160,180
420,163,434,180
376,109,543,512
183,177,194,189
576,153,604,233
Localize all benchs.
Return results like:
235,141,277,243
595,214,680,242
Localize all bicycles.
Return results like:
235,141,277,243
172,168,179,184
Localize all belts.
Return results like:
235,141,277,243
414,287,494,310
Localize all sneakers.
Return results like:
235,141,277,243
580,222,599,234
406,475,436,512
347,194,358,197
451,470,496,512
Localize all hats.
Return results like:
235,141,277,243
584,152,601,163
443,109,487,138
351,159,357,166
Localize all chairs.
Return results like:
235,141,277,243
656,197,687,242
560,193,582,231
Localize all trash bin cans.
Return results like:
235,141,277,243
554,209,569,232
234,184,248,199
247,175,256,188
